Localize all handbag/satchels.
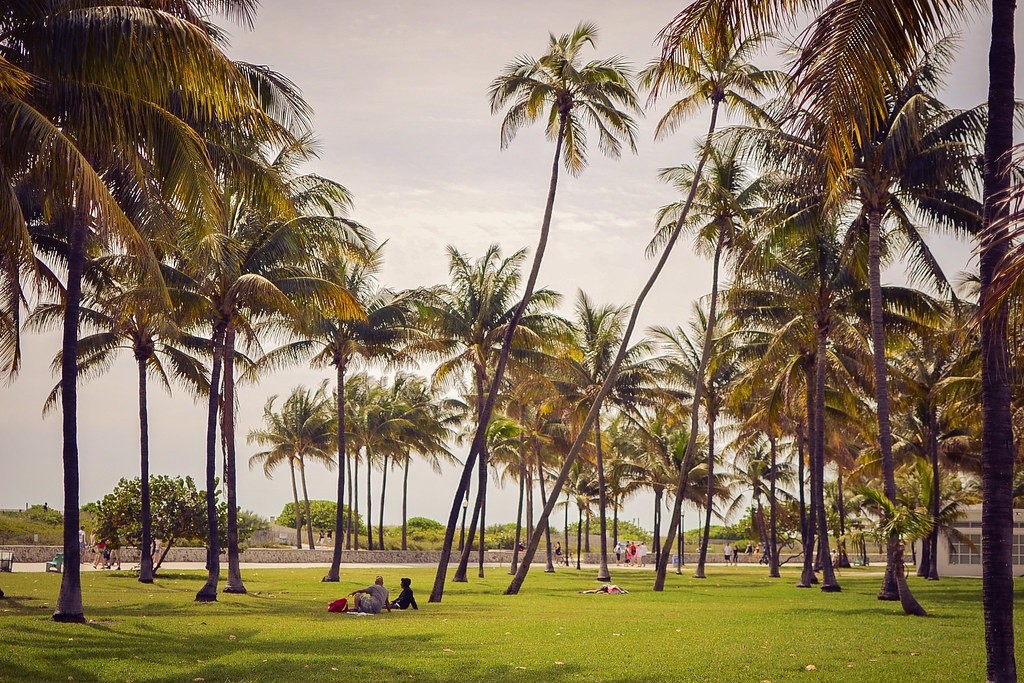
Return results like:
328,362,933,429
327,596,348,612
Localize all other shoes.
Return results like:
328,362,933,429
93,565,97,569
115,567,120,570
100,567,104,569
106,566,110,568
357,611,366,616
346,609,358,614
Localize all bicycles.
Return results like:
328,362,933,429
552,555,564,565
557,552,575,567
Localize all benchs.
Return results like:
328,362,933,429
46,552,65,572
854,557,870,566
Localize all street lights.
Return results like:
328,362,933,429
681,508,685,565
461,498,467,554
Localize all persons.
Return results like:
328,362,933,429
151,539,161,568
763,546,767,561
87,528,121,571
724,542,732,566
327,531,332,546
832,549,841,576
518,542,526,551
745,542,754,562
348,576,393,613
392,578,419,609
623,540,646,567
318,530,325,547
614,542,621,566
79,525,87,563
732,545,739,566
555,541,562,564
43,502,48,511
583,584,629,594
756,544,760,560
